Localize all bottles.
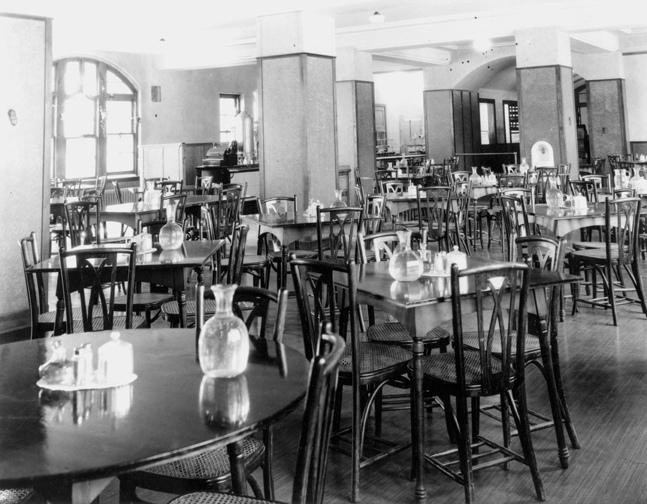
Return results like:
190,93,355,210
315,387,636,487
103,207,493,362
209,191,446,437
97,330,135,380
408,182,416,195
69,389,95,426
196,373,250,437
613,167,646,194
158,248,184,264
310,189,348,217
197,282,248,379
128,225,153,255
100,387,132,427
157,203,183,251
72,342,94,382
518,157,528,175
468,166,496,186
424,275,468,299
420,244,468,274
547,178,587,214
386,162,402,177
388,229,423,282
389,280,421,300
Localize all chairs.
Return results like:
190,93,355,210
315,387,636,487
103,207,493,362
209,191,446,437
16,161,273,341
240,188,582,504
338,148,646,326
117,283,348,504
0,485,38,503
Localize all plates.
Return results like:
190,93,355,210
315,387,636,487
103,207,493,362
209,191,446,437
35,370,138,391
